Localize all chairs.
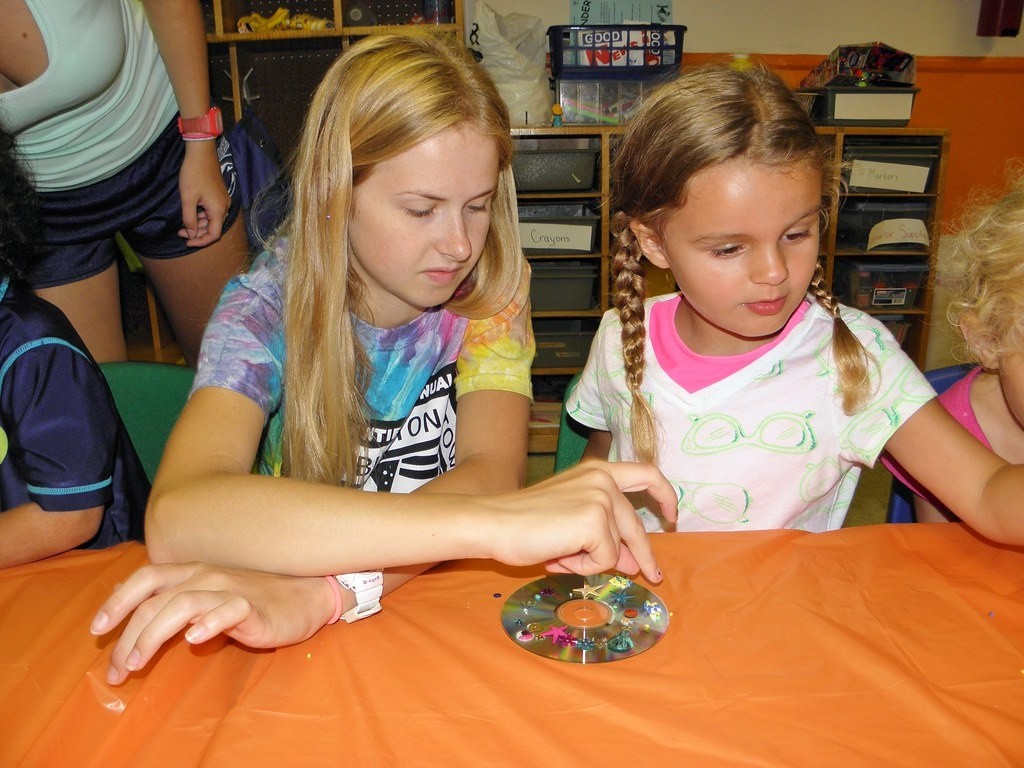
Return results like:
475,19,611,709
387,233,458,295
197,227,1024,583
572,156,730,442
550,370,590,476
887,359,985,523
98,360,195,485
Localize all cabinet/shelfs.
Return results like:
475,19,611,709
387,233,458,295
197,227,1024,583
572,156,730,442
143,0,467,366
499,116,951,451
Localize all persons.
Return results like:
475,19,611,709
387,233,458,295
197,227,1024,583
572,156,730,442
881,174,1024,522
1,123,147,567
90,31,678,686
564,64,1023,546
0,0,248,368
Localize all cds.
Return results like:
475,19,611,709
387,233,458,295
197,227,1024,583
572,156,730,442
500,571,670,664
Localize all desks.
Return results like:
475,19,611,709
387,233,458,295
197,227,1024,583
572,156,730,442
0,520,1024,768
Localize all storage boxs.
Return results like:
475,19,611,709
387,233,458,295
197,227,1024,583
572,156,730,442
793,84,921,128
546,23,687,81
838,201,929,247
835,256,929,311
552,80,658,127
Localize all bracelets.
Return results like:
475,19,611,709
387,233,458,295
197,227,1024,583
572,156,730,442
182,133,217,141
325,575,342,625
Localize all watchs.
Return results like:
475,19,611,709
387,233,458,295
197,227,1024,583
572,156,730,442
178,107,223,136
334,568,385,624
